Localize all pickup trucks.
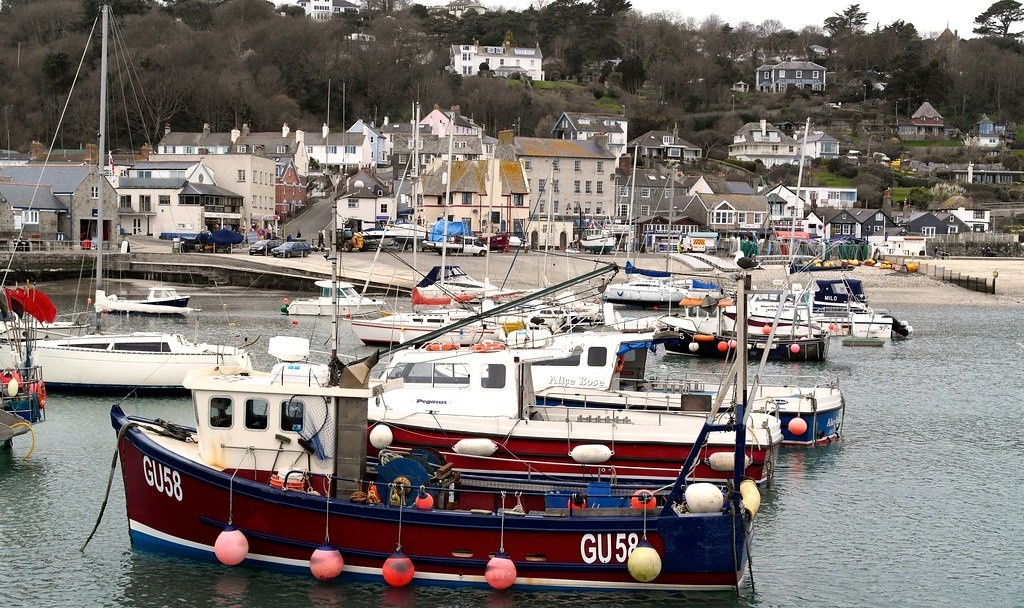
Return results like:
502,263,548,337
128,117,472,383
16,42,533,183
422,236,489,255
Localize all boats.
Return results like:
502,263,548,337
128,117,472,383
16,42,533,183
101,289,200,318
0,6,256,448
479,231,512,251
282,76,388,319
359,218,426,244
347,100,589,347
661,275,914,356
110,320,845,590
598,262,725,307
580,236,617,253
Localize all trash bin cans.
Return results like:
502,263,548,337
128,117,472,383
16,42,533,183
82,240,90,250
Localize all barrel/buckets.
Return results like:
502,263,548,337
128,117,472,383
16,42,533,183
270,467,304,490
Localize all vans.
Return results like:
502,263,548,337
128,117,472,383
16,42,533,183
271,242,312,257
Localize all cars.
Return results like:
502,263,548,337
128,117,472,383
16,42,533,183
564,247,580,254
249,240,283,255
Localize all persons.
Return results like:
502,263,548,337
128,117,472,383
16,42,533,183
934,247,938,257
317,230,326,247
296,230,301,238
911,252,915,260
286,233,292,242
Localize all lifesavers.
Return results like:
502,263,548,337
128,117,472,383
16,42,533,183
36,380,47,408
426,342,460,351
614,354,625,372
470,341,505,352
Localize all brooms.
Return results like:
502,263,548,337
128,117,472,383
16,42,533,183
288,438,316,471
267,433,291,485
